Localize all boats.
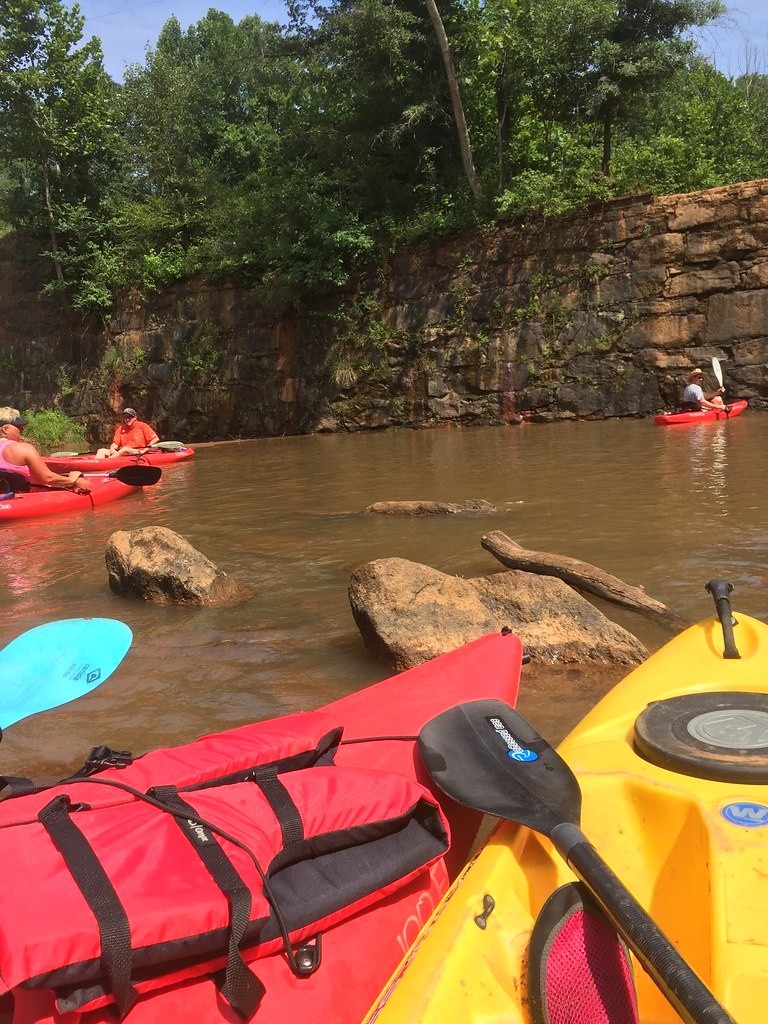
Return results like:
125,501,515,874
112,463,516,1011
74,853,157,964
38,447,194,470
362,577,768,1024
654,396,749,428
0,469,147,522
0,622,522,1023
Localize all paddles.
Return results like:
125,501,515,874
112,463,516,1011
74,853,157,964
49,440,184,458
711,356,728,406
60,464,164,487
415,697,738,1024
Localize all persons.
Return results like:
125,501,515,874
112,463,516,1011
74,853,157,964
0,407,82,492
684,368,726,412
94,408,160,460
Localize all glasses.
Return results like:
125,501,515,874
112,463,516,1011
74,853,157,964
695,376,704,380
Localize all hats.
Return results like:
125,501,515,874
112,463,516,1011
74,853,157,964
0,417,29,430
121,407,137,418
687,368,708,382
